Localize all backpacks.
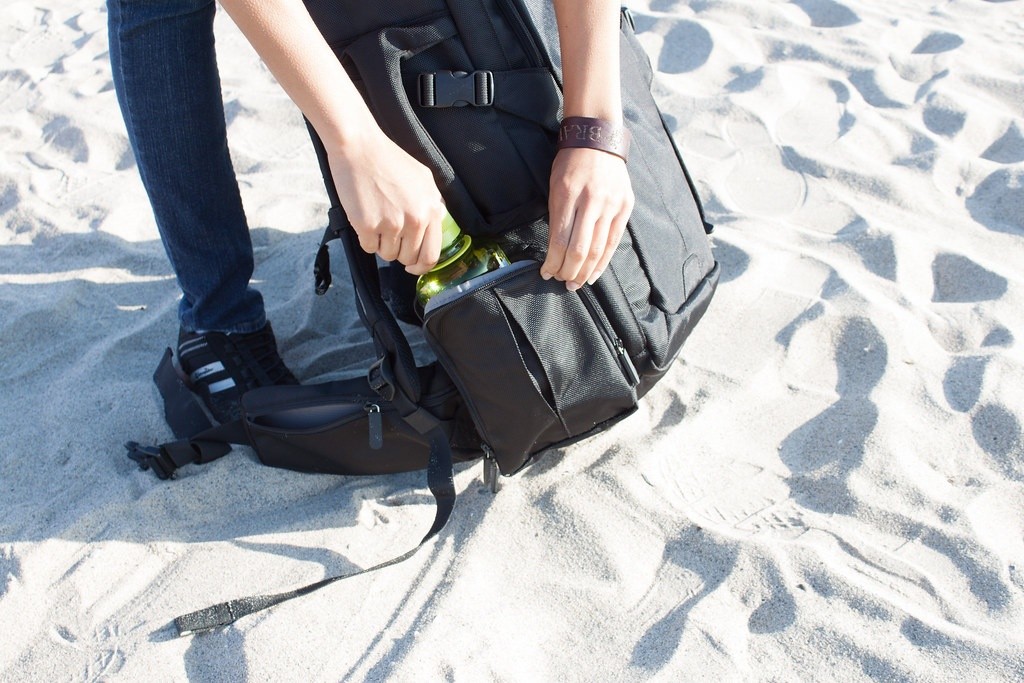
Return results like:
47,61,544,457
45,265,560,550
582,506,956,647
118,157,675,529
294,0,723,549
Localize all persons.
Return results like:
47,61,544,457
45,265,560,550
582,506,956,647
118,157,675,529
107,0,636,431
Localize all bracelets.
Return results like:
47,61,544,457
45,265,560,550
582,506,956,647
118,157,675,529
556,116,632,163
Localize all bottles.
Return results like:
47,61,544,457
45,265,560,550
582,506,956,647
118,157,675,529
414,210,512,313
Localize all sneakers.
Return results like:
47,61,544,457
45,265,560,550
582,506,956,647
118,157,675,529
174,316,302,424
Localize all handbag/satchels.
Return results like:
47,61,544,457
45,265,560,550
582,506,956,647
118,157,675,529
240,357,485,478
